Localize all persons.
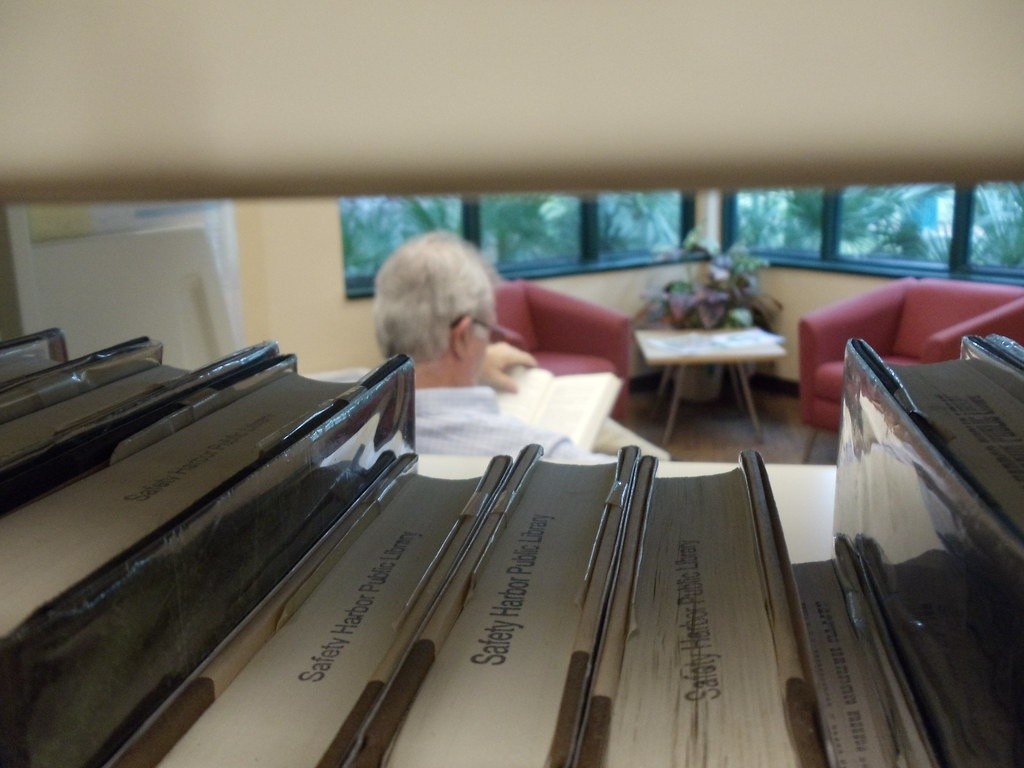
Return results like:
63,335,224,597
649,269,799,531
296,227,593,459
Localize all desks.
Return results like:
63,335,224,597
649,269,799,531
635,325,789,439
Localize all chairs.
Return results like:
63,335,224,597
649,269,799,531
796,277,1024,463
487,280,630,419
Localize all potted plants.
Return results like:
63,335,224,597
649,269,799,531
646,228,782,404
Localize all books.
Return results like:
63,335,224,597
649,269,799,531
418,331,1024,768
0,322,418,768
